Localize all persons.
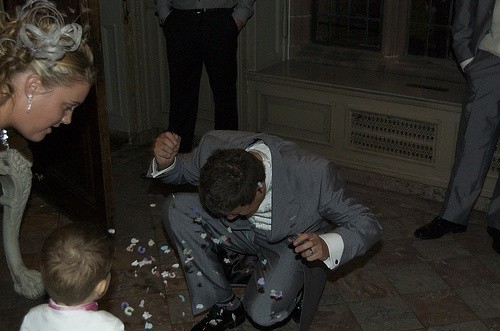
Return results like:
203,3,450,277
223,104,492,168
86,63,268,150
151,0,255,156
0,0,98,142
413,0,500,240
18,222,124,331
144,128,382,331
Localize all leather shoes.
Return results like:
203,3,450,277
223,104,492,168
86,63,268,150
487,226,500,255
191,297,244,331
292,294,304,323
414,217,467,239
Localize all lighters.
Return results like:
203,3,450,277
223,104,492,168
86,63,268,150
290,234,310,253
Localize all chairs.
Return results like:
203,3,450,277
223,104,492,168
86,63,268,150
0,130,45,300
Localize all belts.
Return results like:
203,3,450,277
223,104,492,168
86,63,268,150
191,9,204,15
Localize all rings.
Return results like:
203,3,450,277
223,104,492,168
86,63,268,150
309,248,314,255
